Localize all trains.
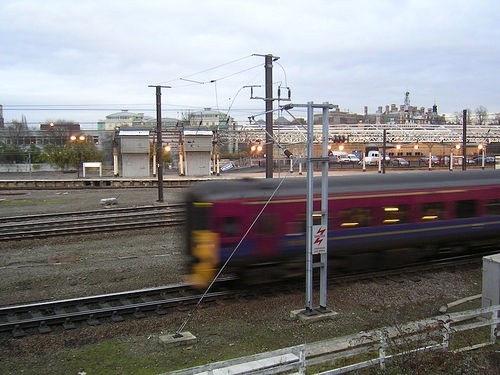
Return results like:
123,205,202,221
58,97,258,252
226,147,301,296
182,167,500,293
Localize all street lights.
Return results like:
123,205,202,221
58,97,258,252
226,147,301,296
70,135,86,178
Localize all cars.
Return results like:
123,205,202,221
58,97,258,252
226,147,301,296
391,158,409,167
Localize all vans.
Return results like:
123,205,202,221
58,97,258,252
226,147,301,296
348,154,360,164
329,151,350,164
368,151,391,165
420,155,440,165
359,157,379,166
